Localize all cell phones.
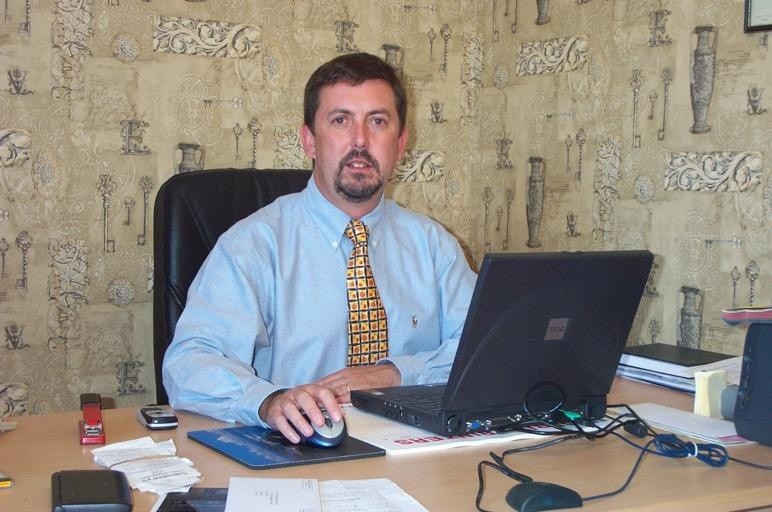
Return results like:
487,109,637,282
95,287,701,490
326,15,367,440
136,408,178,430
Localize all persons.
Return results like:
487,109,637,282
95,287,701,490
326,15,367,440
161,54,478,445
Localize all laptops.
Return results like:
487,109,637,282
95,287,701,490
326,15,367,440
350,251,654,437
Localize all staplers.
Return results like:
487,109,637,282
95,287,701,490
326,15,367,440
79,393,106,445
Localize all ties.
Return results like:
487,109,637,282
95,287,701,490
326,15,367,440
344,219,388,367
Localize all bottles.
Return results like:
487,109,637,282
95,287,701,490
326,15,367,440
533,0,551,25
524,156,546,248
172,142,206,177
687,24,718,134
378,44,407,80
679,285,705,351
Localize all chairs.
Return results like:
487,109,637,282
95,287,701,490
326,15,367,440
149,168,312,403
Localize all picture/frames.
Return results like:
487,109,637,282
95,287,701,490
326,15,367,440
743,0,772,33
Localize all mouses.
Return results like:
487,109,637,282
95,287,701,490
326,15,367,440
295,410,347,448
506,480,582,512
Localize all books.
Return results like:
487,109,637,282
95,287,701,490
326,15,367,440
616,343,740,396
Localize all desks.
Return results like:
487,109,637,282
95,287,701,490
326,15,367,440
0,365,772,510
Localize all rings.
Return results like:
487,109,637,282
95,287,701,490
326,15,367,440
346,384,352,391
